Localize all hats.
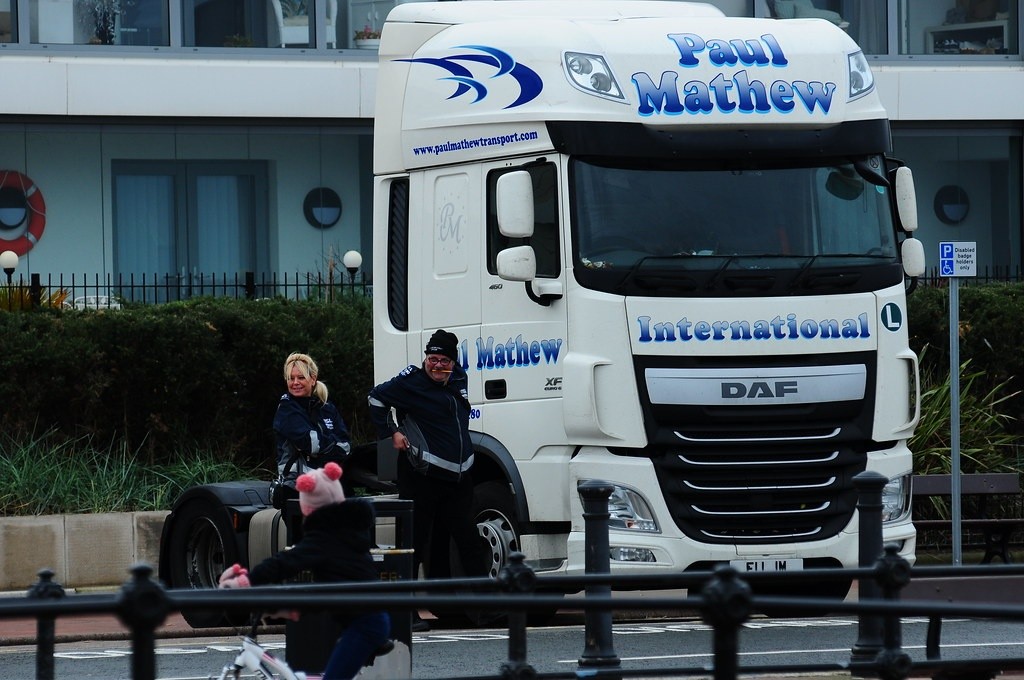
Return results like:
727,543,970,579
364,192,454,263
295,461,345,516
424,329,459,361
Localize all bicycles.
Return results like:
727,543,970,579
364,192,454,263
208,585,308,680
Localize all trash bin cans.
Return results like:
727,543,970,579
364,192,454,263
283,496,413,674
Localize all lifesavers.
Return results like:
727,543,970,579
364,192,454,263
0,169,45,257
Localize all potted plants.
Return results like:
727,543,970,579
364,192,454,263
354,25,381,52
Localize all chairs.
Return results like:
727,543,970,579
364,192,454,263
898,473,1024,610
266,0,339,48
672,188,744,250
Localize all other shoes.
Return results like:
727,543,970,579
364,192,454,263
412,619,430,632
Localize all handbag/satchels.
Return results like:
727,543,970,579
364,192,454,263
268,456,306,508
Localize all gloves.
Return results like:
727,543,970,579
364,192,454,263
219,564,253,591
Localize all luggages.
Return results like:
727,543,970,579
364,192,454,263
247,508,288,572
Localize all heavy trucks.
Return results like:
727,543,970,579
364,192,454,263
157,0,927,635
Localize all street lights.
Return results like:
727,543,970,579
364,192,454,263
0,250,20,313
343,250,362,297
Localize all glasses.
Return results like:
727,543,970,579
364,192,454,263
428,356,453,367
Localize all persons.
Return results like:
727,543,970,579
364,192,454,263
367,329,509,631
274,351,352,548
220,462,389,680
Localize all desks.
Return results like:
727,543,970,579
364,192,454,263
926,20,1011,56
